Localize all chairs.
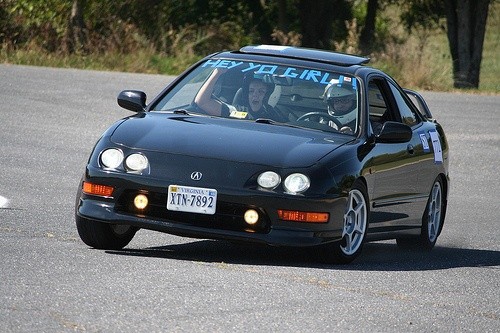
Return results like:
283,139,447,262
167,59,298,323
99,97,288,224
231,88,246,106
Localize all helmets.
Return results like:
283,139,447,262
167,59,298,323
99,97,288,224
242,72,281,112
325,83,357,125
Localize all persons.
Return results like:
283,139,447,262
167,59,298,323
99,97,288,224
193,62,281,122
319,77,357,136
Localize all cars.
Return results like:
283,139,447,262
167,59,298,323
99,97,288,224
74,44,451,266
